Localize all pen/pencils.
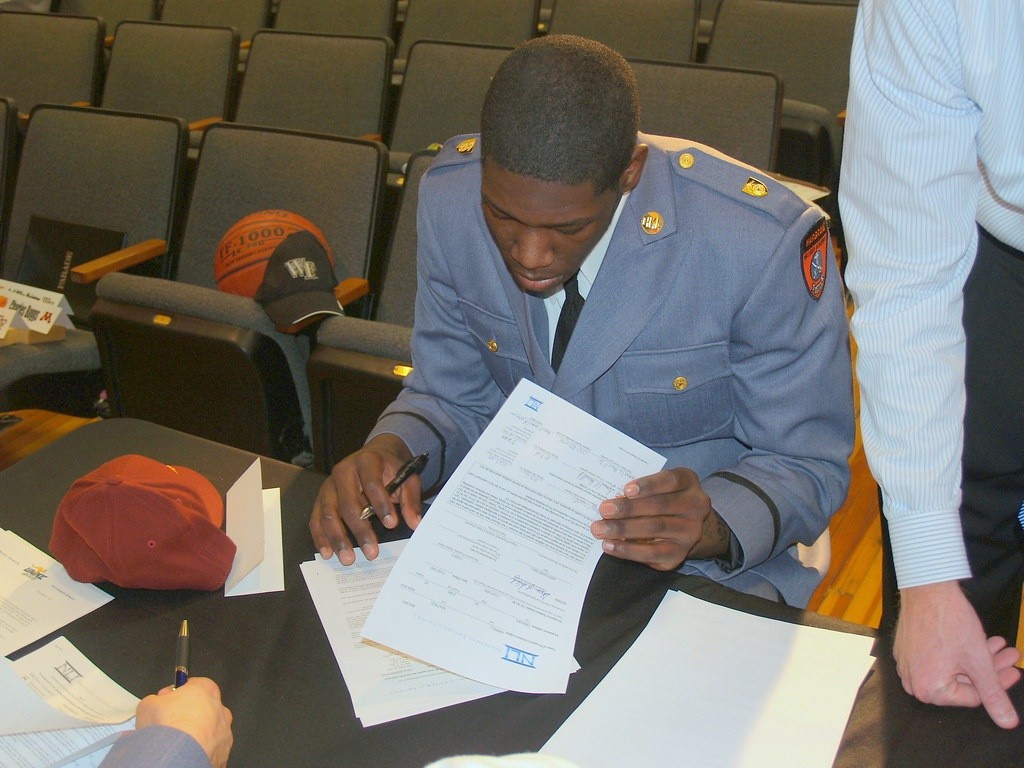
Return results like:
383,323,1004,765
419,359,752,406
359,451,431,520
174,619,189,689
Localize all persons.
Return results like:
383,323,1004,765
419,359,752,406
307,34,856,615
838,0,1024,732
98,675,236,768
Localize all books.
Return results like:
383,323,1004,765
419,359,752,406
0,636,141,768
15,216,125,328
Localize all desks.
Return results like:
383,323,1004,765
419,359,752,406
0,416,1024,768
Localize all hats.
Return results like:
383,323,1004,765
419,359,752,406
50,453,236,591
255,229,346,325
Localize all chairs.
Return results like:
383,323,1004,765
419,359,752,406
0,1,859,478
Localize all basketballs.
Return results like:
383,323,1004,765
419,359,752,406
215,211,335,301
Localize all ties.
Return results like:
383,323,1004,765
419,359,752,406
551,268,585,375
